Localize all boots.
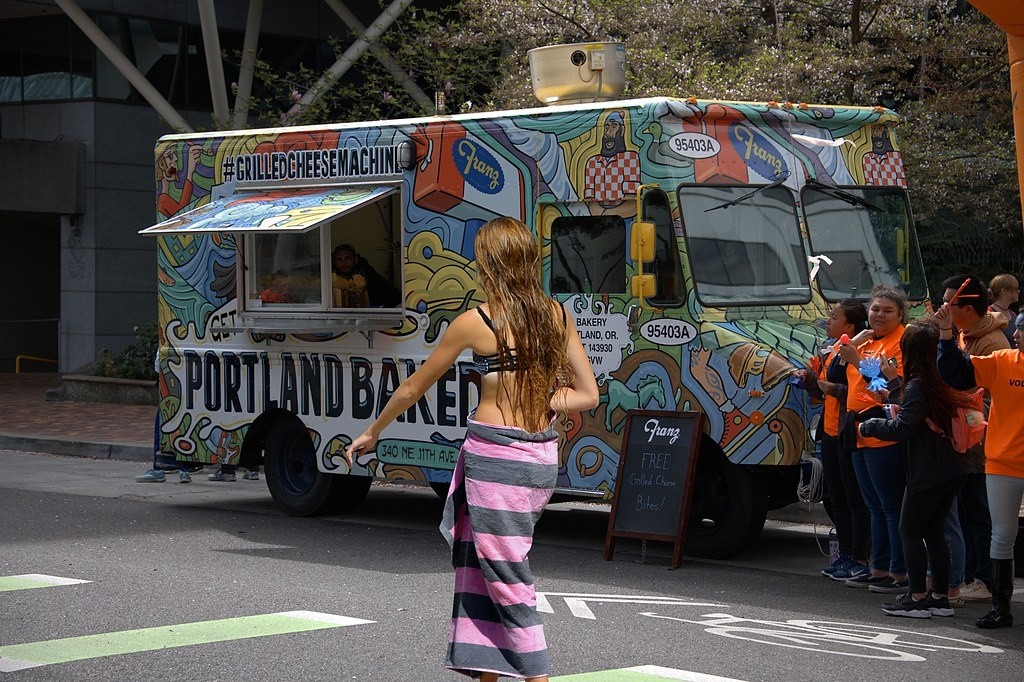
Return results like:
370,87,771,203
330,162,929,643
976,558,1014,629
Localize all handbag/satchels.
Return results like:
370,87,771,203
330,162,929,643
827,526,841,565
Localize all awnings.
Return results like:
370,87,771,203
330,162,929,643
138,186,398,236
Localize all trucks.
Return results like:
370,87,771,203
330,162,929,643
137,42,937,559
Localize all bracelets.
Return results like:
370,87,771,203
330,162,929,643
940,327,952,330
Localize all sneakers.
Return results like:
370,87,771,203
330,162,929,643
868,576,909,592
879,590,932,619
845,573,883,588
242,469,259,479
926,591,954,616
178,470,191,483
133,468,166,482
948,596,965,607
821,555,844,577
958,578,994,600
830,559,872,580
206,470,236,481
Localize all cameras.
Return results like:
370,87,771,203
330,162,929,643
887,357,898,368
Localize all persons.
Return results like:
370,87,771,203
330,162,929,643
261,272,290,302
333,244,390,305
345,216,599,681
793,275,1024,627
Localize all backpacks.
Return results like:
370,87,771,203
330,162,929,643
927,387,987,455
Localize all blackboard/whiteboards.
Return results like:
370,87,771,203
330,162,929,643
607,408,704,541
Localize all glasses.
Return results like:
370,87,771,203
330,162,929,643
940,296,965,307
